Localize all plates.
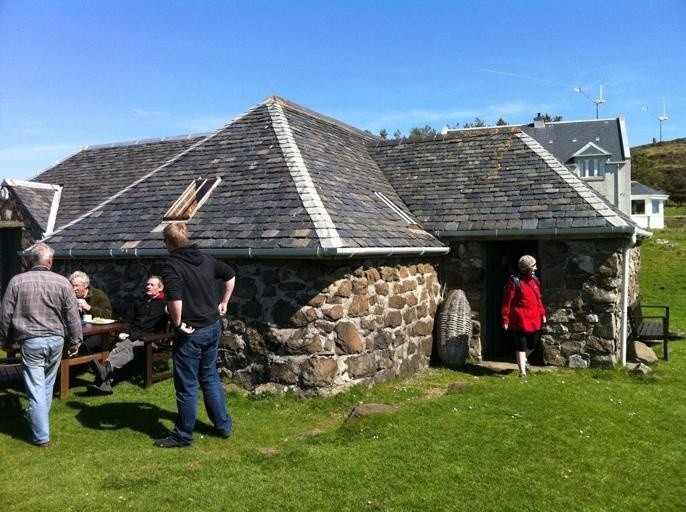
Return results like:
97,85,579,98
82,318,117,326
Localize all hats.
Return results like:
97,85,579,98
518,255,536,270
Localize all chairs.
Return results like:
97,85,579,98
139,313,176,387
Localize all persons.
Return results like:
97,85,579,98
0,244,83,448
87,275,171,395
501,254,546,378
68,270,112,373
154,223,236,447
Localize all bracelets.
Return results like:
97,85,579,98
175,323,182,329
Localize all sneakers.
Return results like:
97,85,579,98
222,432,231,439
155,434,192,448
524,361,531,370
38,443,49,447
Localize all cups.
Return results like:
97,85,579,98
83,315,92,321
77,299,83,309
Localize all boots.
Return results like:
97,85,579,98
87,359,113,395
